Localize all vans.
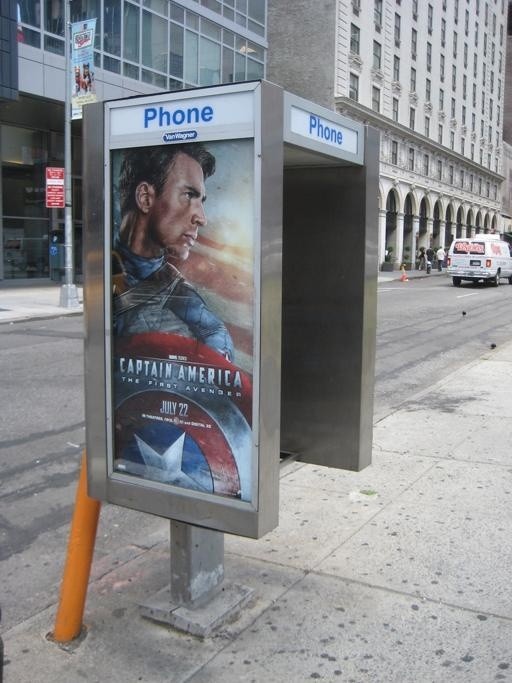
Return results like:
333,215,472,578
446,237,512,286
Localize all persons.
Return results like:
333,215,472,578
417,245,447,272
111,143,234,363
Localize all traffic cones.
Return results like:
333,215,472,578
401,265,407,282
